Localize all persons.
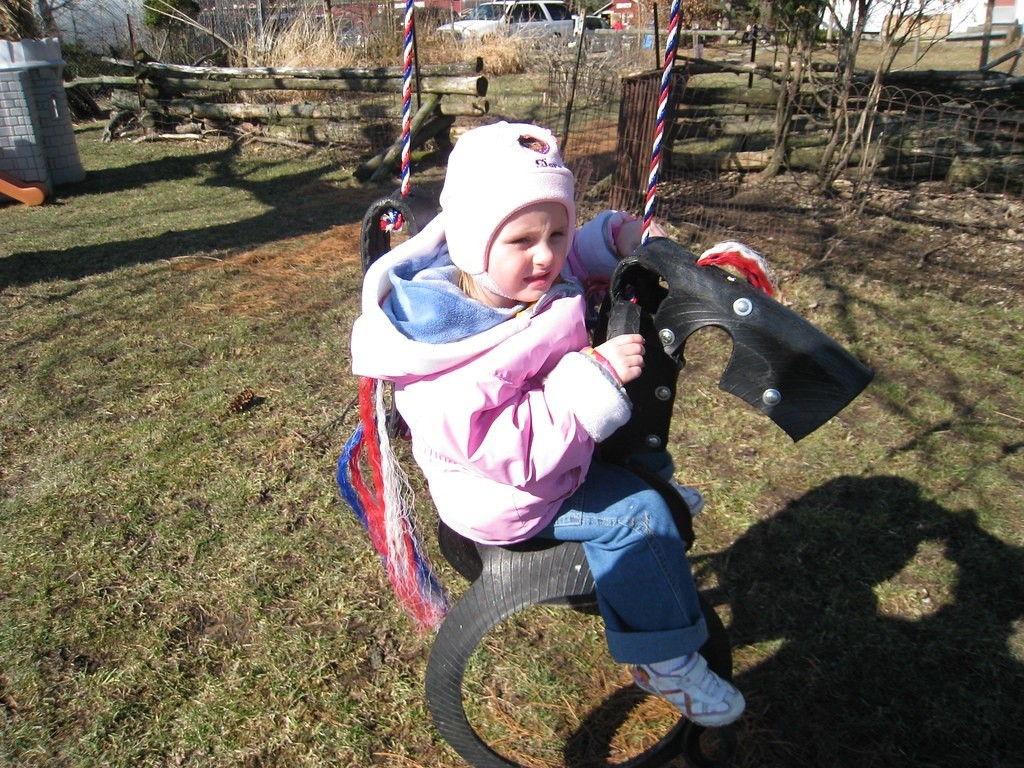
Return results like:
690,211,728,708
350,121,748,728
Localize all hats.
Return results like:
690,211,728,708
439,121,576,274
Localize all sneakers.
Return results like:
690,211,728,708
630,653,746,728
668,479,704,515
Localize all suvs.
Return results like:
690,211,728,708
434,0,575,47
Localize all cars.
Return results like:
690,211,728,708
259,12,367,53
567,13,620,52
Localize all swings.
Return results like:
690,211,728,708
338,0,870,768
